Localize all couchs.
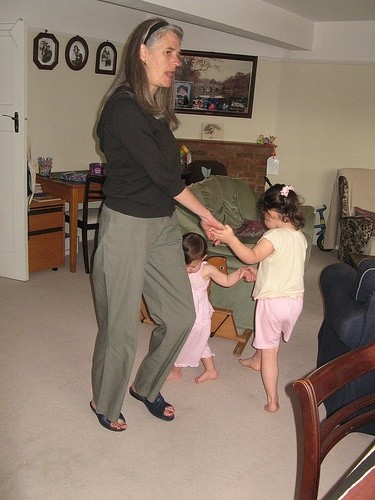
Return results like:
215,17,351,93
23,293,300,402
323,168,375,264
316,258,375,436
140,175,316,357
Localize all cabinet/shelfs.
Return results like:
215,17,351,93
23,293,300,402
28,195,65,273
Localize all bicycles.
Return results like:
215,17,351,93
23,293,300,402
313,204,333,253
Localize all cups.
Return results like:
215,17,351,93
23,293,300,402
39,166,51,176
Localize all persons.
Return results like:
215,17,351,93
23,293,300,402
175,84,190,106
87,16,228,431
101,47,111,67
71,46,83,65
166,232,255,385
39,41,52,64
210,183,308,413
192,94,247,111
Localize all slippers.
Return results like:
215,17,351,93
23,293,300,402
129,386,174,421
90,400,126,431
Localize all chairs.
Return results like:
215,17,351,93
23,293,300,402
64,174,108,273
284,341,375,500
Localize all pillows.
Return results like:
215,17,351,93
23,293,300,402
236,219,267,237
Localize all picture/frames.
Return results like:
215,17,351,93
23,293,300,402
174,49,258,119
95,42,117,75
65,35,88,71
33,32,59,70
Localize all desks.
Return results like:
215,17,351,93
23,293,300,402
36,170,103,273
